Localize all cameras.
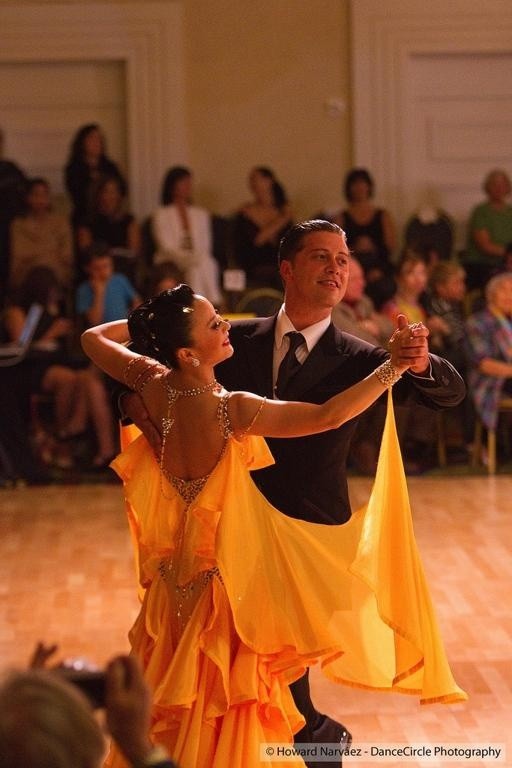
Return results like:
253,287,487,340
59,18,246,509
63,673,117,710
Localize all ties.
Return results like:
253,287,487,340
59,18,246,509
274,332,306,400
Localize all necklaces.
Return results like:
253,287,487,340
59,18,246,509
158,370,226,502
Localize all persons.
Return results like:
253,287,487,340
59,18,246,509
0,121,512,486
213,217,469,767
81,280,470,768
0,638,175,768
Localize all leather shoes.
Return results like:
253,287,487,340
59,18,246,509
298,714,351,768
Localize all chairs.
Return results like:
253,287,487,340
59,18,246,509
415,393,468,472
470,388,512,474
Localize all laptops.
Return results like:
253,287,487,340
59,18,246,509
0,296,48,353
